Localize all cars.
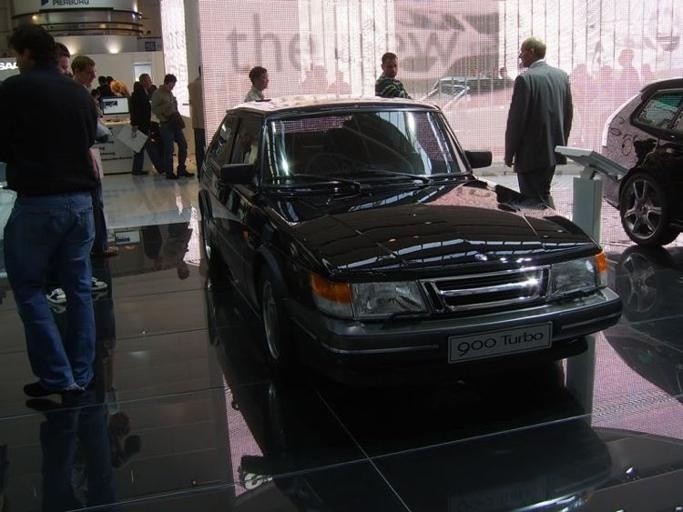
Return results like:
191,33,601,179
194,92,620,386
591,239,683,404
595,77,682,252
195,313,615,511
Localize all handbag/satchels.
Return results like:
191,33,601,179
167,112,185,131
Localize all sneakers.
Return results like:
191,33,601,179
24,379,67,396
105,245,119,254
91,276,108,290
45,287,67,303
131,169,194,180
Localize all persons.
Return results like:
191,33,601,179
504,35,574,211
0,24,97,397
297,60,350,95
96,75,126,98
242,66,270,103
188,64,206,181
567,48,654,153
70,55,118,254
42,41,108,302
141,183,192,280
499,67,515,88
374,52,414,99
24,399,115,512
128,73,196,179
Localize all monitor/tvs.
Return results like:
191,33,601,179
100,95,129,116
114,229,142,247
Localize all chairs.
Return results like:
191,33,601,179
325,127,354,158
356,125,395,163
275,132,301,161
309,155,352,172
